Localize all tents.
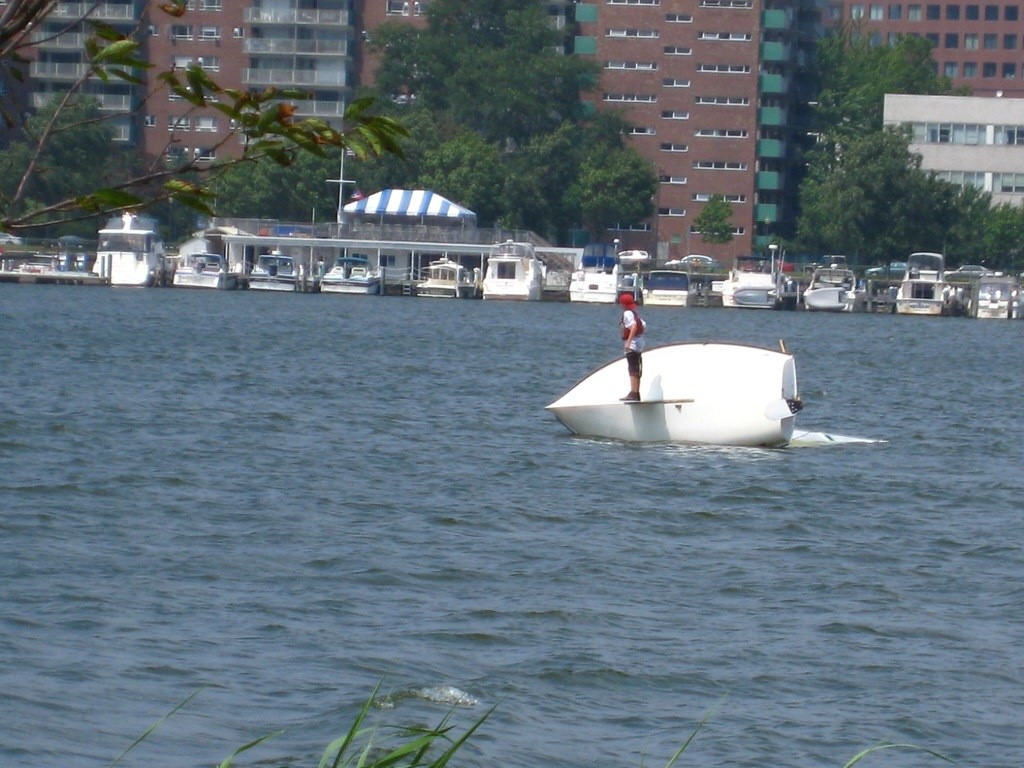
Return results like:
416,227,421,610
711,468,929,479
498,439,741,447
343,188,476,244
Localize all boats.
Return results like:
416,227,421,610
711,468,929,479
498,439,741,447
317,261,382,295
564,263,620,302
803,264,857,312
173,249,235,290
643,271,693,308
896,270,952,317
417,260,466,299
92,212,166,286
722,256,793,309
247,254,303,292
483,240,541,301
543,345,890,449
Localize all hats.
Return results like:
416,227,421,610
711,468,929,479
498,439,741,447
619,294,637,310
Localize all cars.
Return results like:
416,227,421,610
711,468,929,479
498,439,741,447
944,265,1004,283
866,263,908,278
664,255,721,274
619,250,651,268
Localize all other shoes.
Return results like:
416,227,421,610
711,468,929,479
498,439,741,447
619,390,640,401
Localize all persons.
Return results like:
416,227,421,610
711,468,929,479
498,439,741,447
121,211,136,231
619,294,647,401
